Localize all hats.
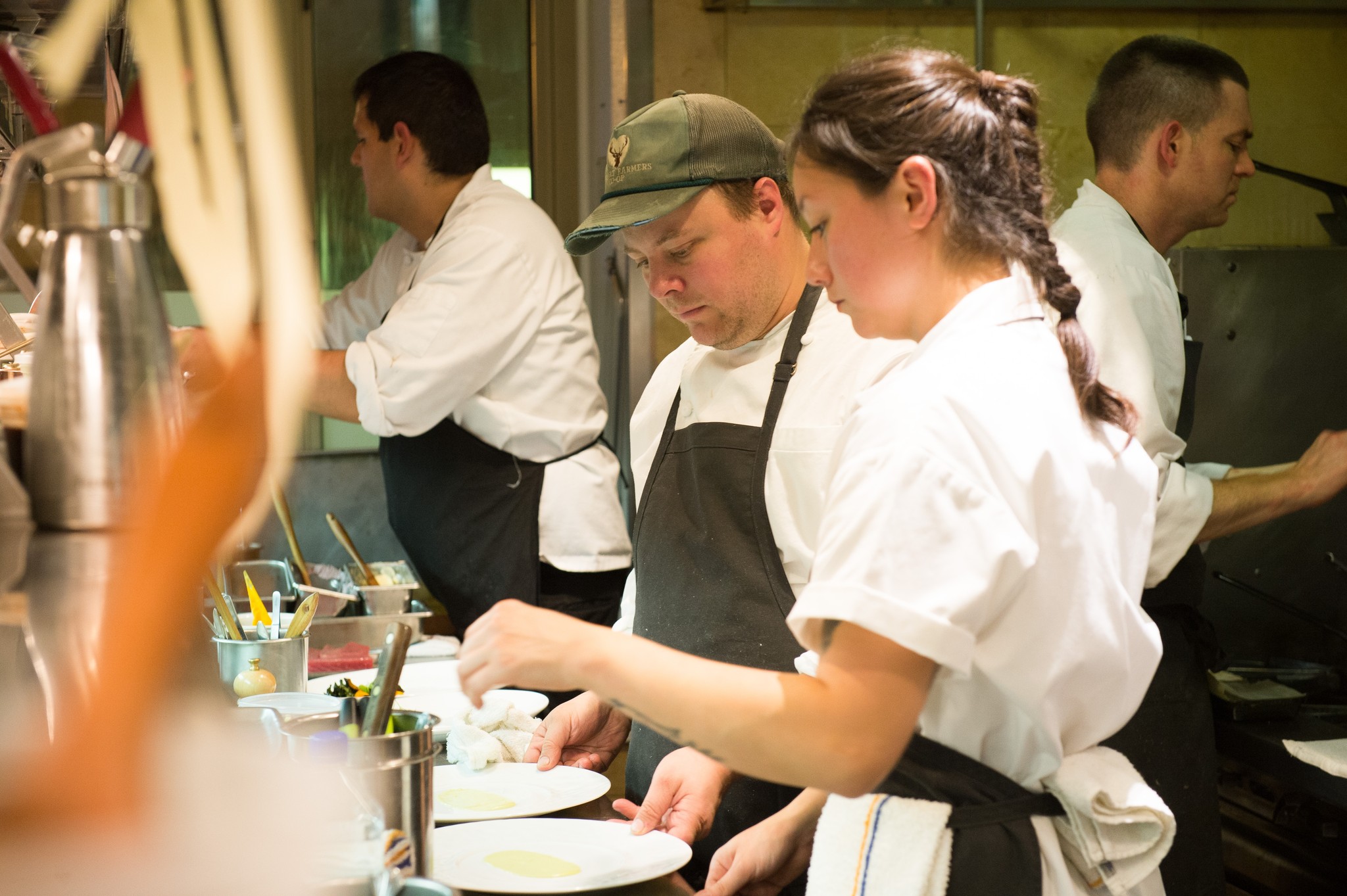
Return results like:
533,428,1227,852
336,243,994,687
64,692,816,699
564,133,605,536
564,89,786,256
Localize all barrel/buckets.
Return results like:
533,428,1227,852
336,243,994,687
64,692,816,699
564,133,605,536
236,692,342,718
212,627,310,691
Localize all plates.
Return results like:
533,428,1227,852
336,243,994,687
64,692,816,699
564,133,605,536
389,690,550,739
1206,651,1336,719
433,764,613,825
305,660,462,695
433,817,694,895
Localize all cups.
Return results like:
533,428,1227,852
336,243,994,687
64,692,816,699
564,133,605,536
279,705,441,878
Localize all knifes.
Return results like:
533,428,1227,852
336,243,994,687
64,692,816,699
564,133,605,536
257,620,270,640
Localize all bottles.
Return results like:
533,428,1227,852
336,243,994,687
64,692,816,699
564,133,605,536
0,313,38,427
27,166,182,529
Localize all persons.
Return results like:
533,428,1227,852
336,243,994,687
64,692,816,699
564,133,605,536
169,51,634,821
523,88,918,896
456,48,1164,895
1046,32,1347,895
